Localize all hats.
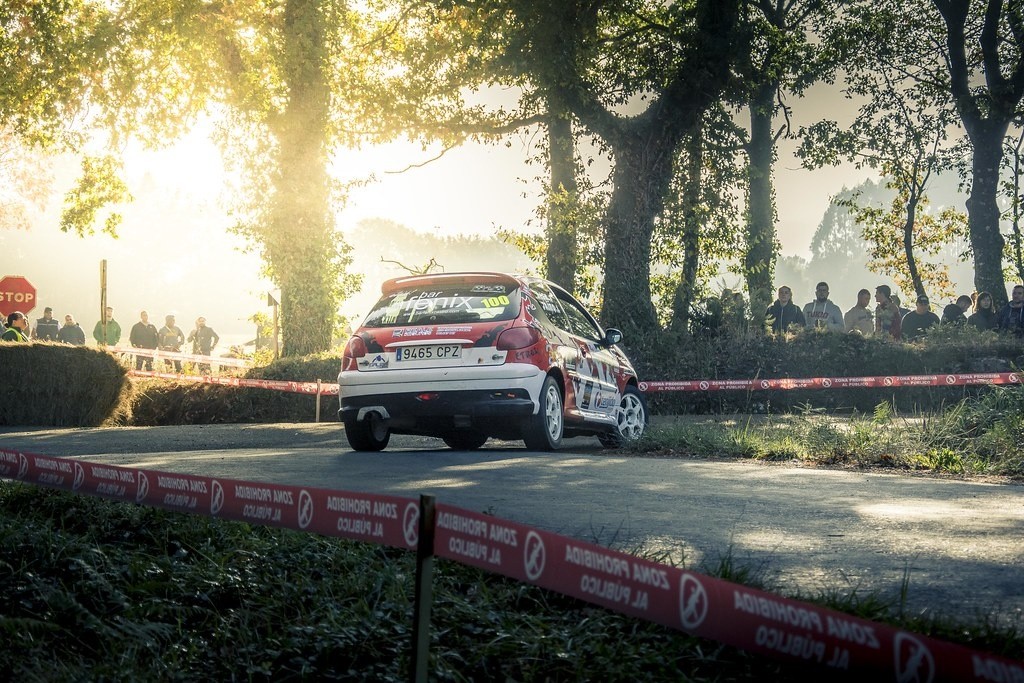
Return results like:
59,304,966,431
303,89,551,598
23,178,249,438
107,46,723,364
917,294,929,305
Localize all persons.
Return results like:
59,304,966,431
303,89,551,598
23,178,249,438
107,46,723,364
705,285,1024,338
0,306,220,375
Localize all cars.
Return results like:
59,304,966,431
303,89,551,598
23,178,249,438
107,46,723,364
335,271,650,454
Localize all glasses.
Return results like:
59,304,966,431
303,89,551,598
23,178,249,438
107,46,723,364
18,316,24,319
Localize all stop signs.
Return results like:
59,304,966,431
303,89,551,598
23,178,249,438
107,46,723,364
0,275,37,319
